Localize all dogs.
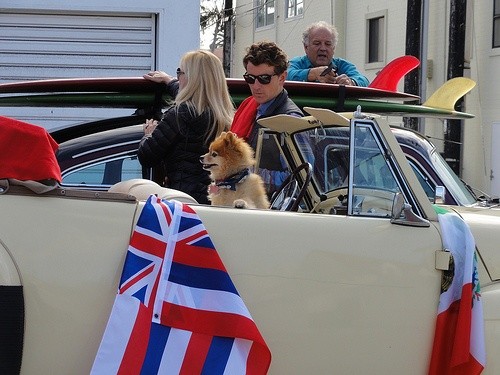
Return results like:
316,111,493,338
199,131,270,211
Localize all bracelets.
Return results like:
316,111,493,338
352,79,357,86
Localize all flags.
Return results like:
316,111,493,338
428,203,487,375
89,193,271,375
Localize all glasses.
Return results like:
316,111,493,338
319,62,337,77
177,68,184,79
242,71,278,84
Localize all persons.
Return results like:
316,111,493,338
285,21,370,88
137,49,236,205
231,41,315,200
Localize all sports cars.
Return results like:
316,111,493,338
0,106,500,375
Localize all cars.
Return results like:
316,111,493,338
45,115,499,210
